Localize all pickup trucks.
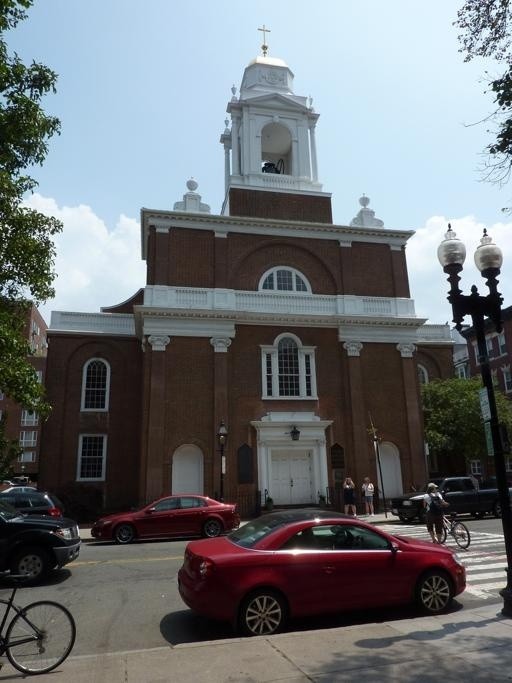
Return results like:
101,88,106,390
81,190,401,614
389,473,511,521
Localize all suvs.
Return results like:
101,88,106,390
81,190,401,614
1,476,67,523
0,498,81,583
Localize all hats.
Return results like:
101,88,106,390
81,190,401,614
427,483,438,488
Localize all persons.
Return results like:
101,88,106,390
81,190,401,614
422,483,450,544
361,477,375,517
343,473,357,517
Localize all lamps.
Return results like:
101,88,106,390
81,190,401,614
290,425,300,440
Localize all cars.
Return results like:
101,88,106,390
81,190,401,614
174,509,469,638
91,492,241,545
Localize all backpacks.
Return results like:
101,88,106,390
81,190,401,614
429,496,441,514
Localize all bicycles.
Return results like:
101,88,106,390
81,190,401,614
1,570,76,677
426,503,471,549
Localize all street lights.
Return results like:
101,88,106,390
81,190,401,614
215,418,230,503
367,424,387,518
438,222,511,616
